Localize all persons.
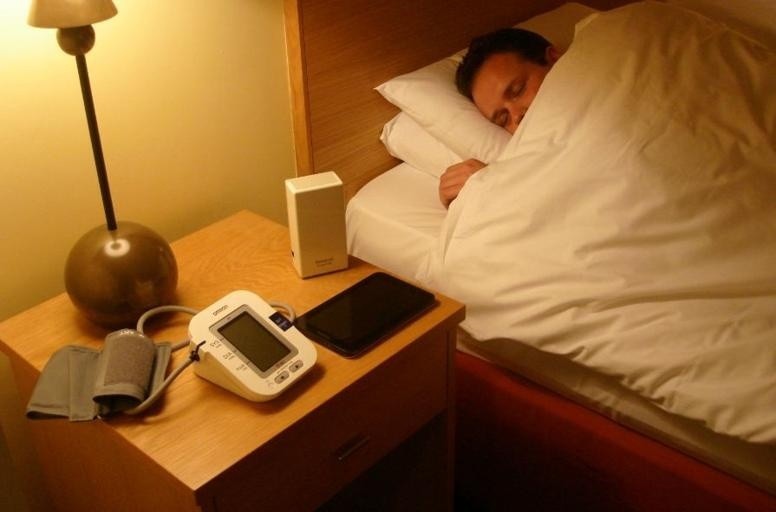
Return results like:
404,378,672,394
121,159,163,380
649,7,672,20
434,24,567,211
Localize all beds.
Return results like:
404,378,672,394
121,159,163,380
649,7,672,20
283,0,776,512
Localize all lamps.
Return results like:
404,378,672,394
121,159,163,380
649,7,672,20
27,0,178,335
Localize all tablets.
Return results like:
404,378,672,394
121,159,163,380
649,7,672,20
293,271,435,359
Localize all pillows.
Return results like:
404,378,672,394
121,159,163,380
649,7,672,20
373,3,602,165
379,112,465,180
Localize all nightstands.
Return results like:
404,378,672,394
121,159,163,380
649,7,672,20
0,210,466,512
